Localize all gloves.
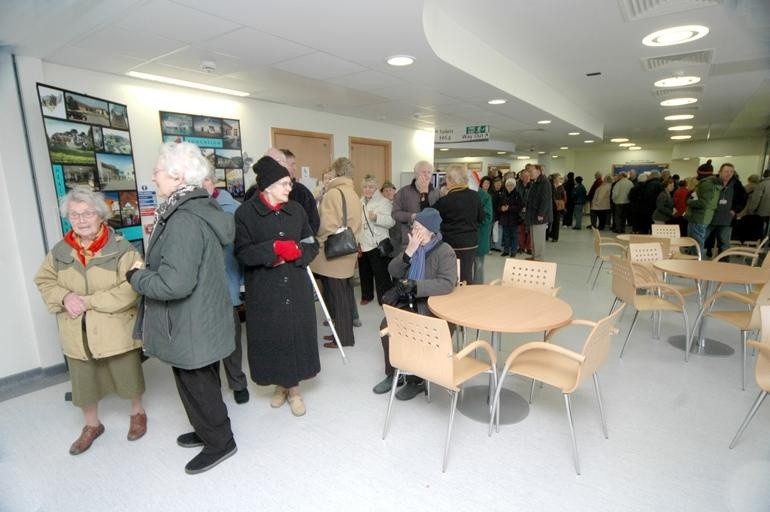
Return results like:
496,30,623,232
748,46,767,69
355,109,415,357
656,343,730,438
381,281,406,306
274,240,302,266
400,279,416,296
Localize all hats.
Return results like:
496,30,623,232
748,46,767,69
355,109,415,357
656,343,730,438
253,156,291,192
489,167,502,179
697,159,713,176
380,181,396,193
415,208,442,234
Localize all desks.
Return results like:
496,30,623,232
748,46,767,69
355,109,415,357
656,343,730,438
651,259,770,358
427,285,573,425
616,234,696,246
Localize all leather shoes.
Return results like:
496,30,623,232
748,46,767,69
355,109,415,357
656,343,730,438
610,227,625,233
490,248,501,251
720,256,729,262
572,227,581,230
361,299,369,304
127,409,147,441
324,335,335,340
706,250,712,257
510,253,516,257
630,231,641,234
517,249,532,254
552,239,558,242
69,420,105,455
525,257,544,262
598,227,604,230
546,235,549,240
586,225,596,228
501,253,509,256
324,342,337,348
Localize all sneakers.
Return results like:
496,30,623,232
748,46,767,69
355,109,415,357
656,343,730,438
373,374,404,394
177,432,205,447
271,385,287,408
234,388,249,403
561,225,573,229
287,394,306,417
185,442,237,474
395,381,426,401
488,251,492,255
352,319,362,327
324,319,335,326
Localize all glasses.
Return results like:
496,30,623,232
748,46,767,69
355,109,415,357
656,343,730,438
444,175,449,179
66,211,100,219
153,168,168,176
383,188,395,193
411,226,427,230
274,182,293,187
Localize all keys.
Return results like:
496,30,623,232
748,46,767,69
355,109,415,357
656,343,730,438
523,166,551,262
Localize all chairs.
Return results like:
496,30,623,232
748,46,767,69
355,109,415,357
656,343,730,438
627,235,671,298
708,236,769,313
652,224,702,295
628,241,702,340
586,226,628,290
710,250,769,312
488,302,627,476
729,305,770,450
609,254,689,362
453,259,466,353
382,304,499,474
474,257,562,388
684,278,770,391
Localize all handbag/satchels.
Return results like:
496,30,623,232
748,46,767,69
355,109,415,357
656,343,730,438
363,205,394,257
324,188,358,260
675,190,687,217
554,195,565,210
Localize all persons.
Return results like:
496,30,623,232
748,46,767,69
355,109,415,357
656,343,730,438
391,161,444,252
611,173,633,234
478,176,494,194
672,181,690,217
245,149,319,238
280,149,297,180
587,172,604,231
563,172,577,229
652,181,689,239
373,207,457,401
707,163,746,263
317,157,363,346
592,174,613,231
316,170,336,214
629,174,646,234
492,167,516,180
546,173,566,242
429,165,482,285
500,179,520,258
360,175,396,306
575,174,588,230
126,141,236,474
203,165,250,406
233,157,322,417
661,170,681,195
539,164,549,180
518,171,534,255
748,169,770,241
611,168,639,183
638,171,662,236
34,186,145,455
728,170,750,258
379,180,398,203
685,160,719,259
488,179,506,252
745,174,760,195
470,170,492,284
